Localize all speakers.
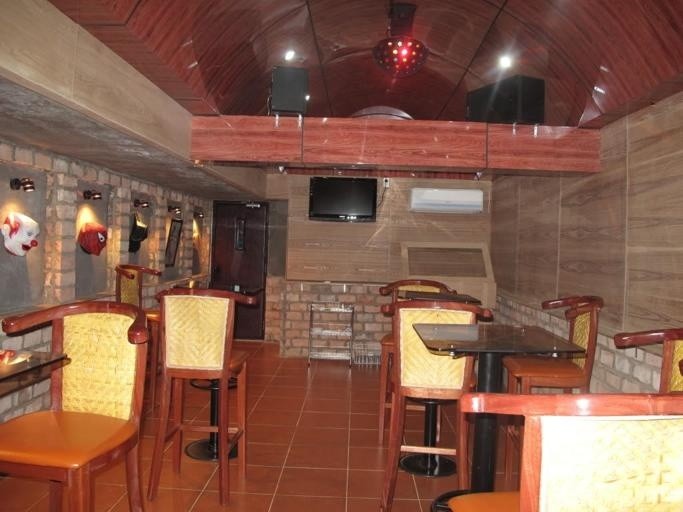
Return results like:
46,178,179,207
465,75,545,123
271,65,308,113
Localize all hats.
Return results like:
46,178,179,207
129,213,148,252
1,213,40,256
79,223,107,256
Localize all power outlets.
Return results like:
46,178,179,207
383,178,389,187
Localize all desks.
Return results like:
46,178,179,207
395,288,483,307
411,319,586,491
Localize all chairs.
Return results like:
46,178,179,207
503,293,603,394
376,279,458,443
2,300,155,510
615,326,683,394
452,385,683,511
382,300,492,508
115,263,165,385
150,289,254,506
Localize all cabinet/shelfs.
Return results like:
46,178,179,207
307,303,354,368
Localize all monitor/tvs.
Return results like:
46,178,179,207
308,176,377,222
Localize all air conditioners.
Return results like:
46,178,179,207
407,187,482,215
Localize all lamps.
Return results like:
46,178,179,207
374,3,429,79
9,177,204,219
